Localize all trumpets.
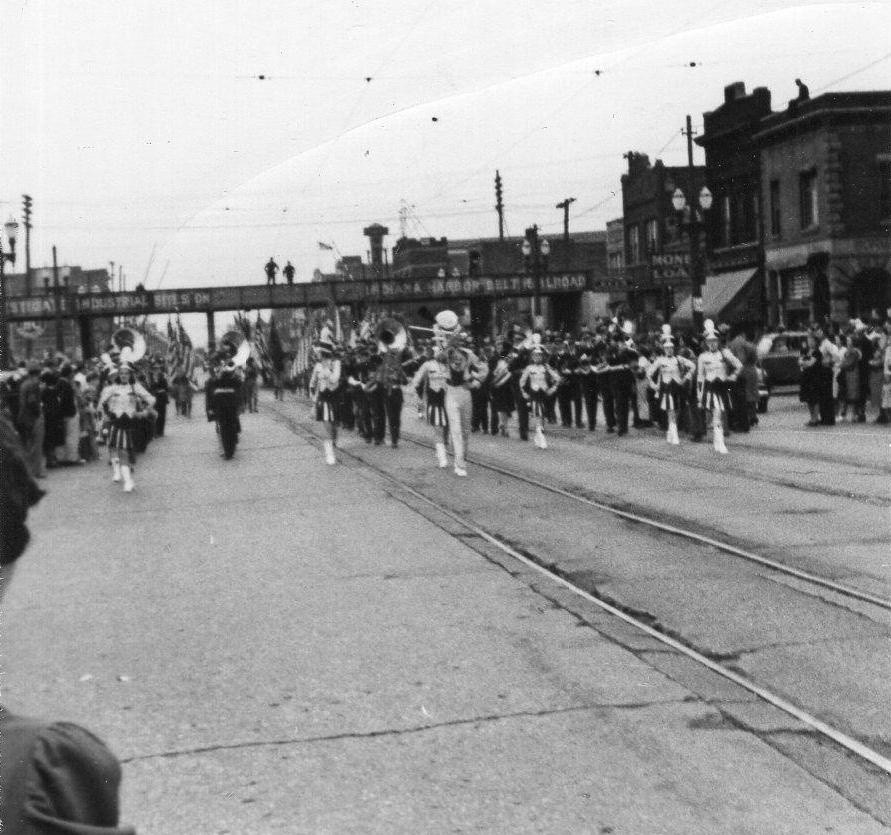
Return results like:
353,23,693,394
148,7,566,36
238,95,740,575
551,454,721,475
225,359,236,372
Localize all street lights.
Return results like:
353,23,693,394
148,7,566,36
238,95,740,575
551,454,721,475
520,223,552,331
673,180,714,342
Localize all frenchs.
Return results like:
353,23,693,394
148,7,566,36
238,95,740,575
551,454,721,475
615,301,638,369
112,328,146,362
375,318,407,354
219,331,250,366
491,318,532,387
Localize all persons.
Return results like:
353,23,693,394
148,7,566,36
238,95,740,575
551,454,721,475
0,255,891,489
0,404,147,834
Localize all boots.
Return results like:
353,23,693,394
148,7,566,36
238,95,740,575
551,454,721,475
111,457,121,483
666,423,681,445
713,428,729,453
122,464,135,492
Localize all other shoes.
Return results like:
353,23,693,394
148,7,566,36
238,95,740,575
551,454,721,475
435,442,450,468
535,416,548,449
454,455,468,476
323,439,336,464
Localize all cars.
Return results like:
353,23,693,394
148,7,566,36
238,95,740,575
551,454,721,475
753,329,810,413
